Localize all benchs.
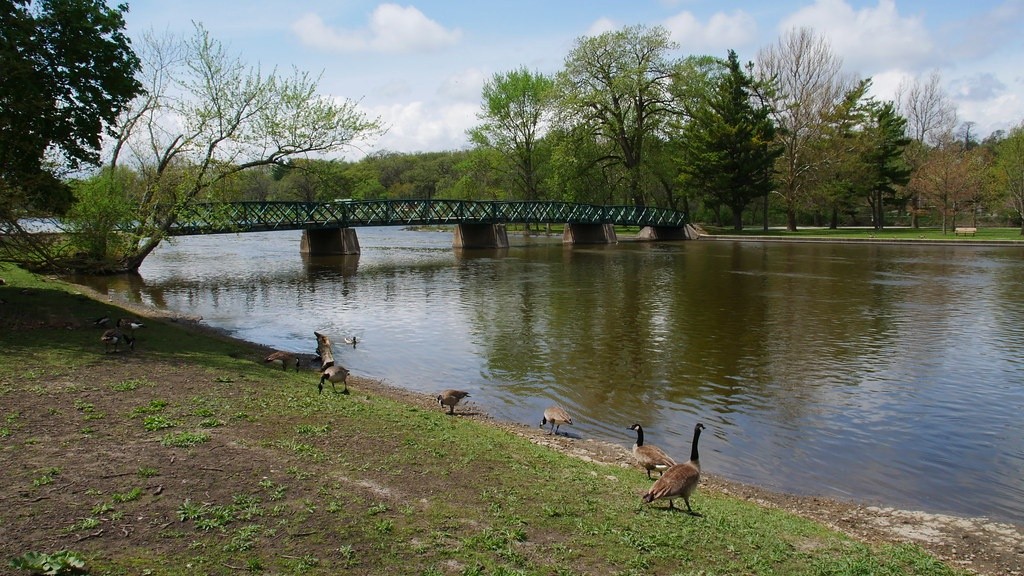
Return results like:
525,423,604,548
955,227,977,236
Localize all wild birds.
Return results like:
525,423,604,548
616,241,619,244
93,311,146,353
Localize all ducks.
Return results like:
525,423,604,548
539,407,573,432
643,423,710,516
263,351,300,373
627,424,676,478
345,336,356,343
437,389,471,413
318,365,350,394
